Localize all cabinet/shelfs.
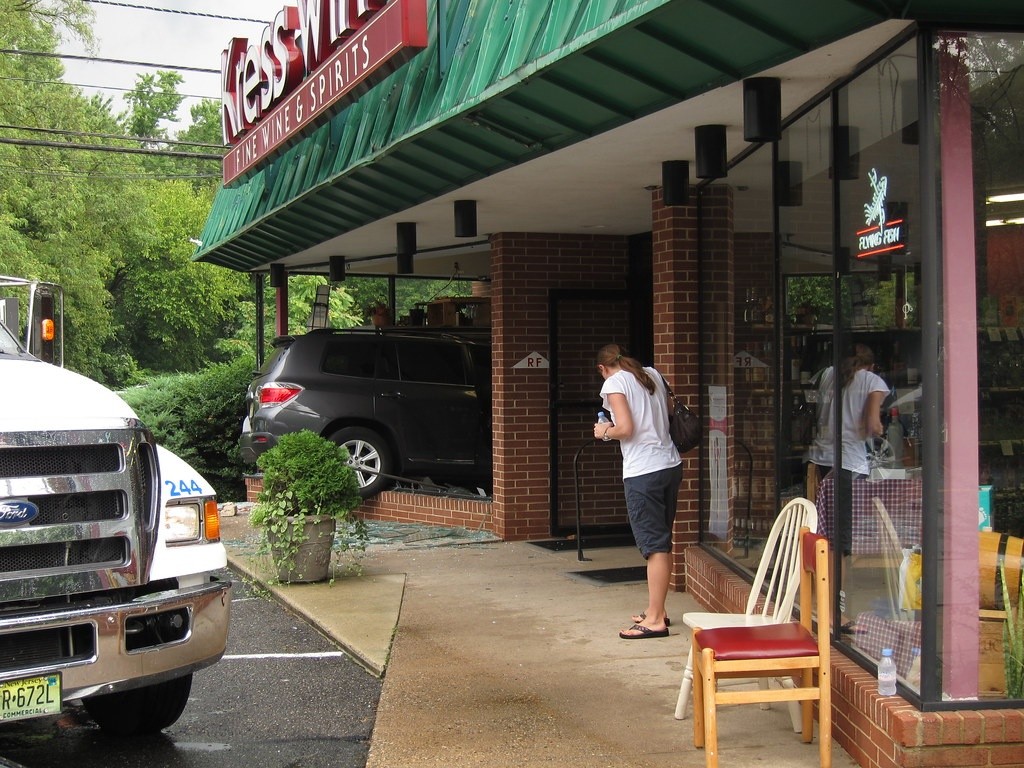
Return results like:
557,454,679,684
736,323,924,496
976,329,1024,536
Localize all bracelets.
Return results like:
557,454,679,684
602,427,611,441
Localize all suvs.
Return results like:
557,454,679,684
240,327,492,499
0,279,232,737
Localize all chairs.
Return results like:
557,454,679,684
675,497,818,738
693,525,834,768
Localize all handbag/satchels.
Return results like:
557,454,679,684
790,366,828,446
657,368,702,454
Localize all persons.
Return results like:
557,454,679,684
593,343,683,640
801,342,891,502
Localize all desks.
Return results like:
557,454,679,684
816,467,927,557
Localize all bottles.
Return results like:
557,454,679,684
877,648,897,696
733,287,832,547
887,407,904,468
598,412,611,441
905,647,921,688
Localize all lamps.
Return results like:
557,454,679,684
330,256,345,282
742,76,782,142
775,162,803,207
660,161,691,208
397,253,415,274
454,200,478,238
695,124,727,178
829,125,861,181
270,264,284,288
902,81,920,149
396,222,417,255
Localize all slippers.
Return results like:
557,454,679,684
619,624,669,639
635,612,670,626
841,621,868,635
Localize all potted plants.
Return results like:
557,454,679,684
253,428,368,586
363,297,392,326
